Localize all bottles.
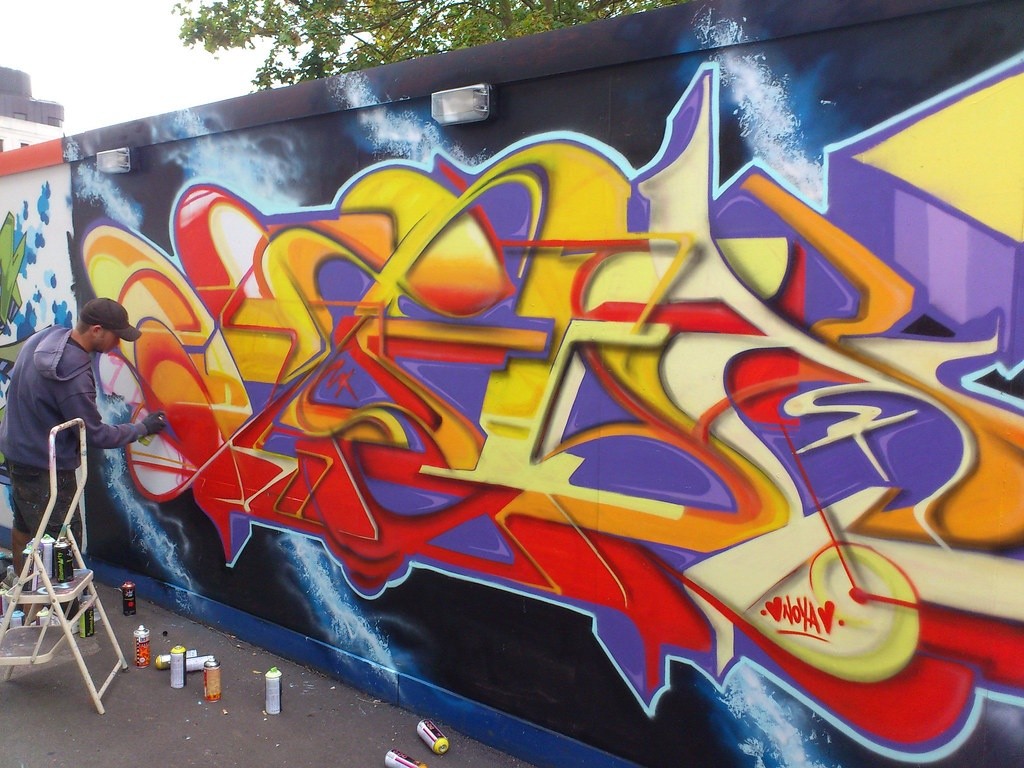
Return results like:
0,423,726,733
170,645,187,688
138,414,163,446
123,582,136,616
80,602,94,638
417,718,449,754
265,667,282,715
134,624,150,669
186,654,215,671
203,656,221,703
385,749,427,768
22,535,74,593
155,649,197,669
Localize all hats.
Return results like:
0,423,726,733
80,297,142,342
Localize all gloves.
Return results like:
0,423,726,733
142,411,167,435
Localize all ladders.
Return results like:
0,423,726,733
0,417,129,715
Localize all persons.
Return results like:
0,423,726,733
0,298,168,635
